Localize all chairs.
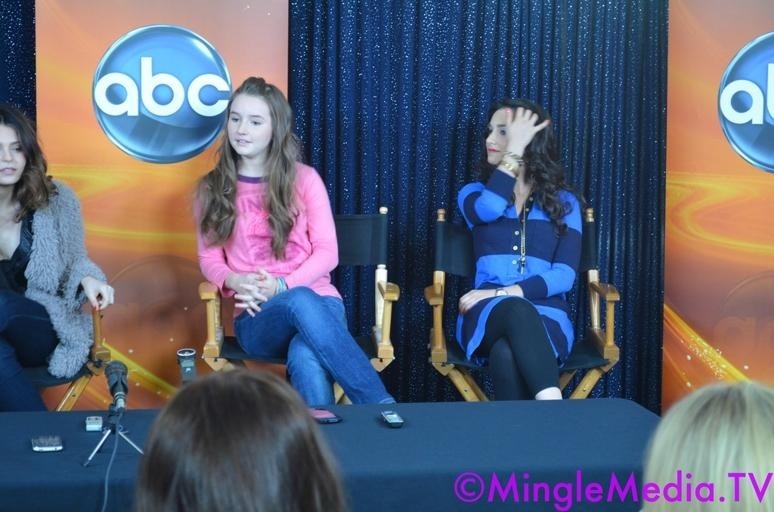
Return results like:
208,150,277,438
24,304,111,411
424,208,621,402
198,205,400,406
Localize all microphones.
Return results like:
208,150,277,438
105,359,129,413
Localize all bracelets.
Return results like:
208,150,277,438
275,274,285,294
497,159,521,179
501,150,524,166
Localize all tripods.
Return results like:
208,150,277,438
83,412,144,467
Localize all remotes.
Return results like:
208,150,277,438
85,415,103,432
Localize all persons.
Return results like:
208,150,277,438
0,98,117,412
129,366,345,512
455,100,584,402
192,74,396,406
636,377,773,511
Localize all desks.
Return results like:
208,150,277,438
0,399,661,512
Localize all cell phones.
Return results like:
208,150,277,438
307,406,344,424
380,409,405,428
30,434,64,452
